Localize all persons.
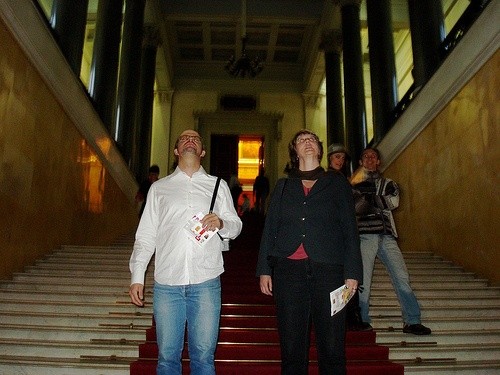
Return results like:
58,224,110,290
128,128,431,375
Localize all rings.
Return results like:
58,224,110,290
352,287,356,290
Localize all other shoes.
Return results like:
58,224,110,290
403,321,431,335
362,321,373,331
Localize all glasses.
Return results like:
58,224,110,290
179,134,201,142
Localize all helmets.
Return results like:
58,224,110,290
327,144,346,155
